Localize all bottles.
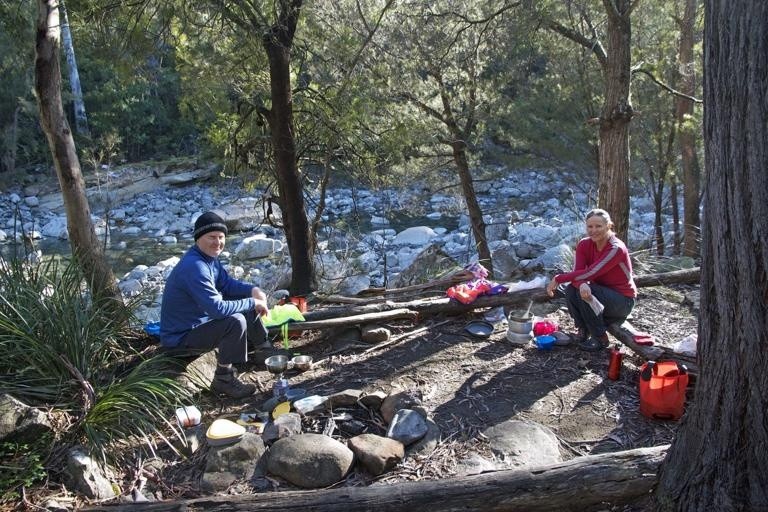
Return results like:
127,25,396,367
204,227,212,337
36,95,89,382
609,343,620,381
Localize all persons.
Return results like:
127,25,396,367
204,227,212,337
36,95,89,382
546,208,637,351
160,212,290,399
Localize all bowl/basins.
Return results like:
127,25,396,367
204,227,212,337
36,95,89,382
291,355,313,372
464,320,494,337
264,355,289,373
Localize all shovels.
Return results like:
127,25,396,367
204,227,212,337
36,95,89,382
357,271,474,296
522,300,533,319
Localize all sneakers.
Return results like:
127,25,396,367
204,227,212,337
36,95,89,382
210,370,256,399
573,326,591,343
580,332,610,350
254,347,289,365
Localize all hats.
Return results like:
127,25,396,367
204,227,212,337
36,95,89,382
191,212,228,242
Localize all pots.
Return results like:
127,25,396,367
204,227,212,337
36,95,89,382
507,310,535,333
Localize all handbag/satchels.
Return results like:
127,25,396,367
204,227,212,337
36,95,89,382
640,358,689,420
